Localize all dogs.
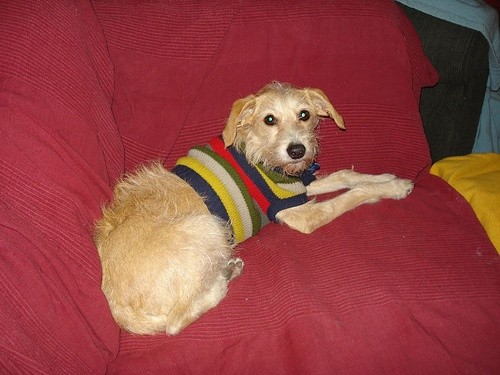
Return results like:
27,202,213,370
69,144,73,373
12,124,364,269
93,79,416,336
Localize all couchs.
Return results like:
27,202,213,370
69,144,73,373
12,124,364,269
0,0,500,375
